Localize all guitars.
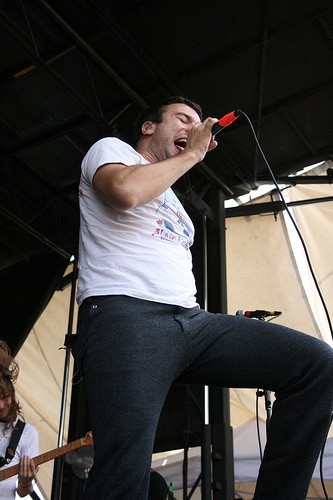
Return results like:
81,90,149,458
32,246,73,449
0,431,93,483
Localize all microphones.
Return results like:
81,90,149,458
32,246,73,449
211,109,242,138
236,309,282,319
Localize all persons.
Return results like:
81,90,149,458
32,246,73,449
76,97,333,500
0,341,40,500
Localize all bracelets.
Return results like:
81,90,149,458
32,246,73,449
20,482,32,487
